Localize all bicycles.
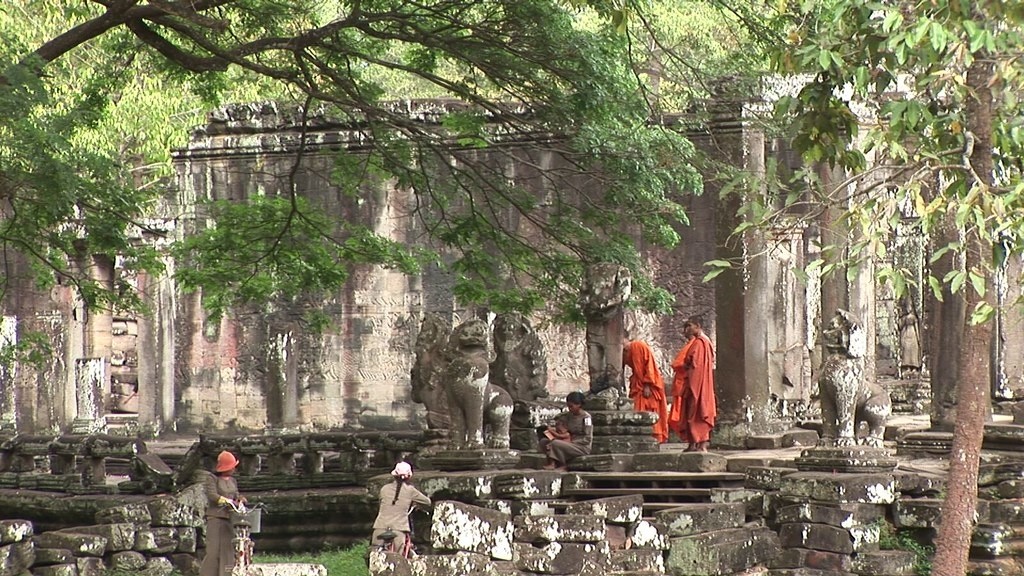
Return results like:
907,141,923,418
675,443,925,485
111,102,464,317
216,498,269,576
376,503,430,555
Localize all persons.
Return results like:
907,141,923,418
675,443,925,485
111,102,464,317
540,391,593,472
898,304,921,372
199,450,248,576
671,315,716,452
623,329,668,444
371,462,431,558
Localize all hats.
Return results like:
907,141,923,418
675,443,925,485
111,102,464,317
216,450,240,474
391,462,413,480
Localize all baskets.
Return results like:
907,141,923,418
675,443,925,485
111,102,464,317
409,517,415,535
228,508,261,534
